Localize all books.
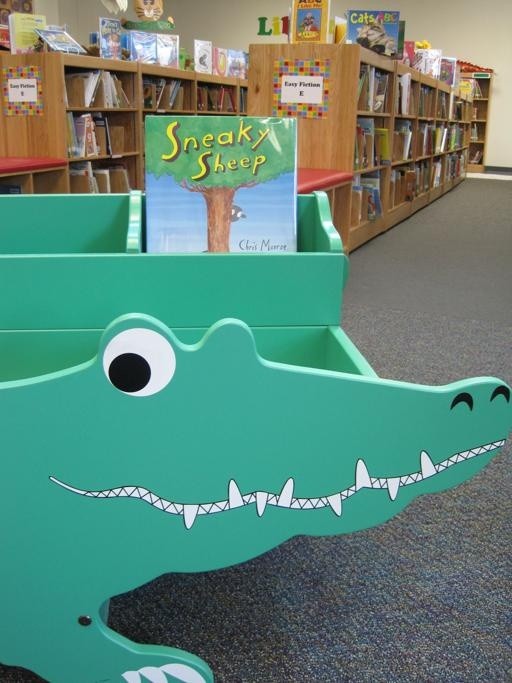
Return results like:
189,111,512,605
291,0,405,59
403,41,460,87
141,112,300,254
66,69,246,194
7,10,248,77
353,66,483,219
